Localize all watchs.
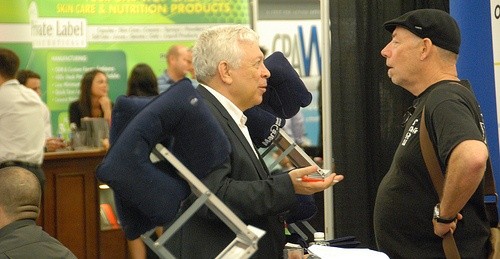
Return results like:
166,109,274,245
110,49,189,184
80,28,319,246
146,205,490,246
433,204,458,223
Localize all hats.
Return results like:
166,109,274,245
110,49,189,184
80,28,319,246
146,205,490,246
383,10,461,54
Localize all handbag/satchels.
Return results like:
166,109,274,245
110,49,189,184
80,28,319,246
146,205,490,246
489,227,500,259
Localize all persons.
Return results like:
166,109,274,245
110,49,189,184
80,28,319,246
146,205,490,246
259,46,306,147
0,167,77,259
0,48,48,184
124,63,163,259
69,68,114,148
16,69,66,152
156,44,188,94
163,26,344,259
373,9,492,259
183,45,199,89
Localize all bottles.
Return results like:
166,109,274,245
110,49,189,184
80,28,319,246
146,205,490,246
308,232,329,259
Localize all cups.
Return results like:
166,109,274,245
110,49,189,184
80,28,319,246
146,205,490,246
80,117,100,148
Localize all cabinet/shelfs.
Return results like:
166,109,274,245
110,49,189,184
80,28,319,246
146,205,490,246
37,149,125,259
